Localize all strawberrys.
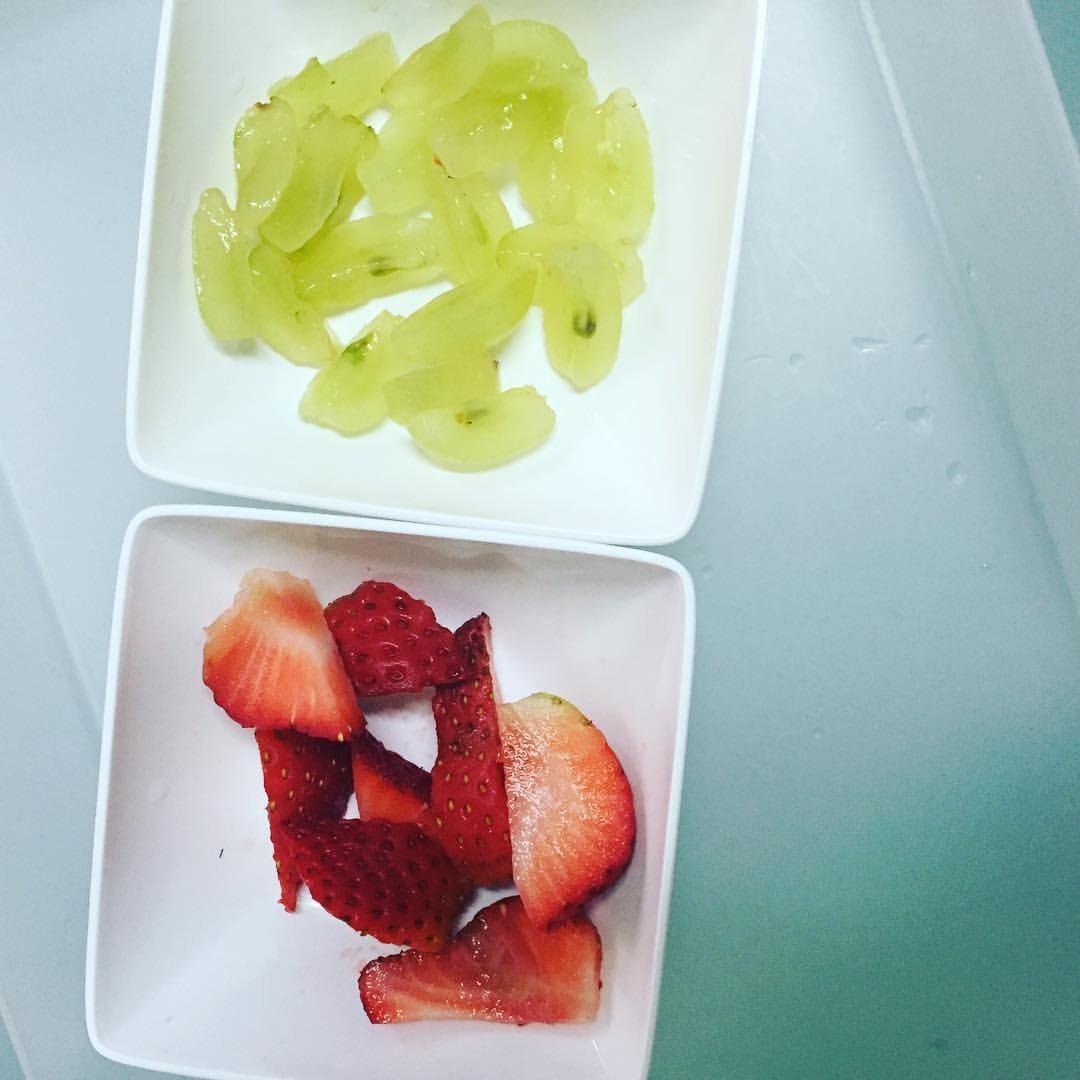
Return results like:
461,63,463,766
203,564,639,1025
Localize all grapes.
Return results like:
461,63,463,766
190,4,656,473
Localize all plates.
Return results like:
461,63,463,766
123,0,767,547
83,501,698,1080
